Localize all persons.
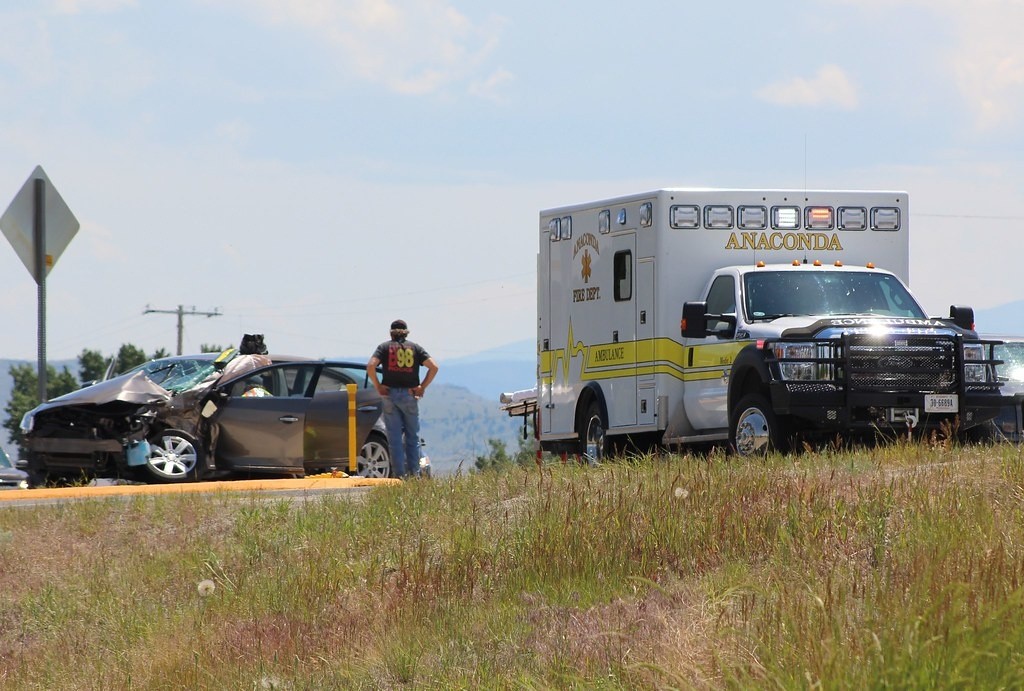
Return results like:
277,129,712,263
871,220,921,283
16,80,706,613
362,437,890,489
367,320,438,478
239,375,273,396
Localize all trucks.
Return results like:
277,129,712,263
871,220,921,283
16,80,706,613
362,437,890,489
532,186,967,461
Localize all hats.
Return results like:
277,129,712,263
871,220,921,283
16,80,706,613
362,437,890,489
247,375,263,384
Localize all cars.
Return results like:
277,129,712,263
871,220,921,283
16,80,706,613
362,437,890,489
19,348,430,488
965,335,1024,443
